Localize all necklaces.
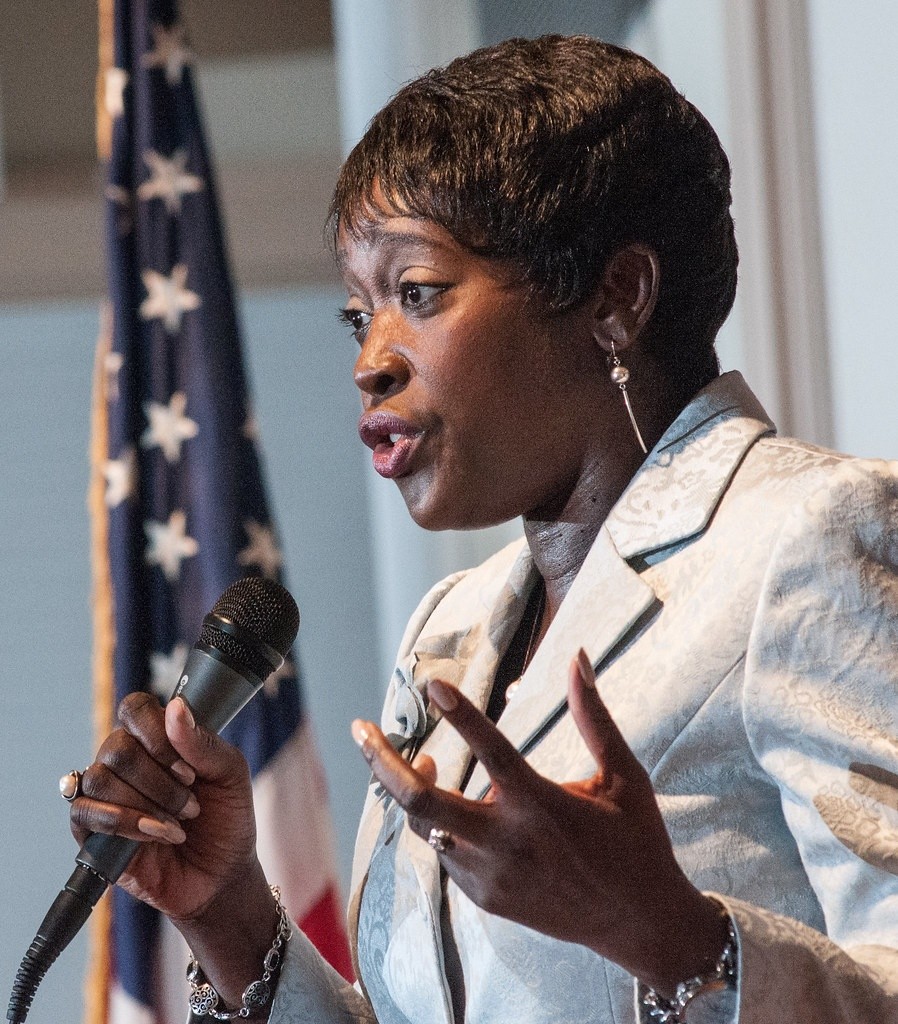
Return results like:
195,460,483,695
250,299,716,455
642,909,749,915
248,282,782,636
516,580,549,677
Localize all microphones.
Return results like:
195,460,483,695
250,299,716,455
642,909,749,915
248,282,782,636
6,576,301,1024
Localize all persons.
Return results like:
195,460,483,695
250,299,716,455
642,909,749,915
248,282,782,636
68,31,896,1024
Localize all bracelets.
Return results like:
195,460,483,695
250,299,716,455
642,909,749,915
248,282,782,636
184,880,293,1024
643,908,739,1024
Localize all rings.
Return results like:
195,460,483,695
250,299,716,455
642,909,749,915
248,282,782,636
429,825,451,854
58,768,83,802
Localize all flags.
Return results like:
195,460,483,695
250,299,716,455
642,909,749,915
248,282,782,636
104,2,369,1024
